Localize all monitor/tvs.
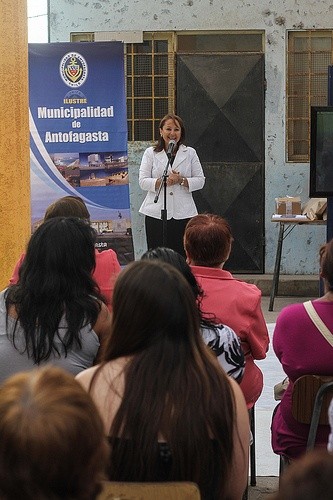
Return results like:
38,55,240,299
309,105,333,198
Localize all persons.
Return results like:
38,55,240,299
75,258,250,500
10,196,122,311
142,247,245,385
183,213,270,409
278,447,333,500
138,115,205,257
271,240,333,460
0,217,113,382
0,367,108,500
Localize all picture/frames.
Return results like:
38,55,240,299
309,106,333,198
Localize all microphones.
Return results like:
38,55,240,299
167,139,176,156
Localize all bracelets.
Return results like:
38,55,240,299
181,178,184,186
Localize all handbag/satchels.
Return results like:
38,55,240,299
274,378,289,401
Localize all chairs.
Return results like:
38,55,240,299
278,374,333,489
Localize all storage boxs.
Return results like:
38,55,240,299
275,197,301,217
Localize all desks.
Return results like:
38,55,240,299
268,214,327,311
